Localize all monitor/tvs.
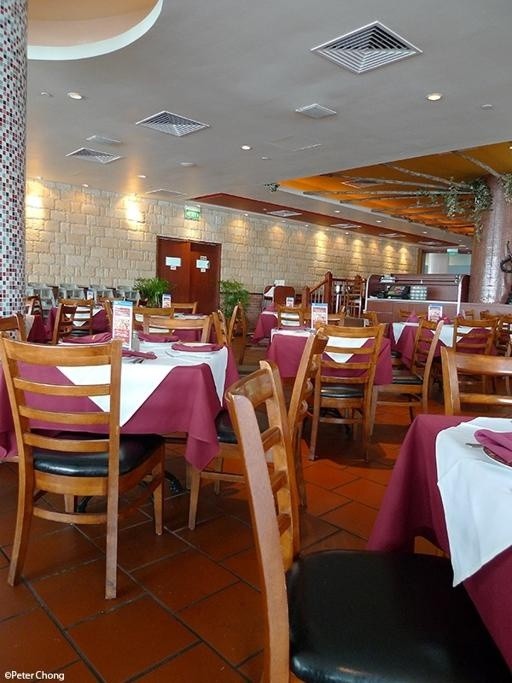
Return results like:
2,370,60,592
386,284,408,299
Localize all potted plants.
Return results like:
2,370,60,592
132,275,169,308
217,278,251,336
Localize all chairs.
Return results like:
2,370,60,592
0,286,512,682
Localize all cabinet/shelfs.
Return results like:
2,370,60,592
364,274,471,322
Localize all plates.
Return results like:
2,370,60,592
481,445,512,470
165,346,216,358
57,339,108,346
